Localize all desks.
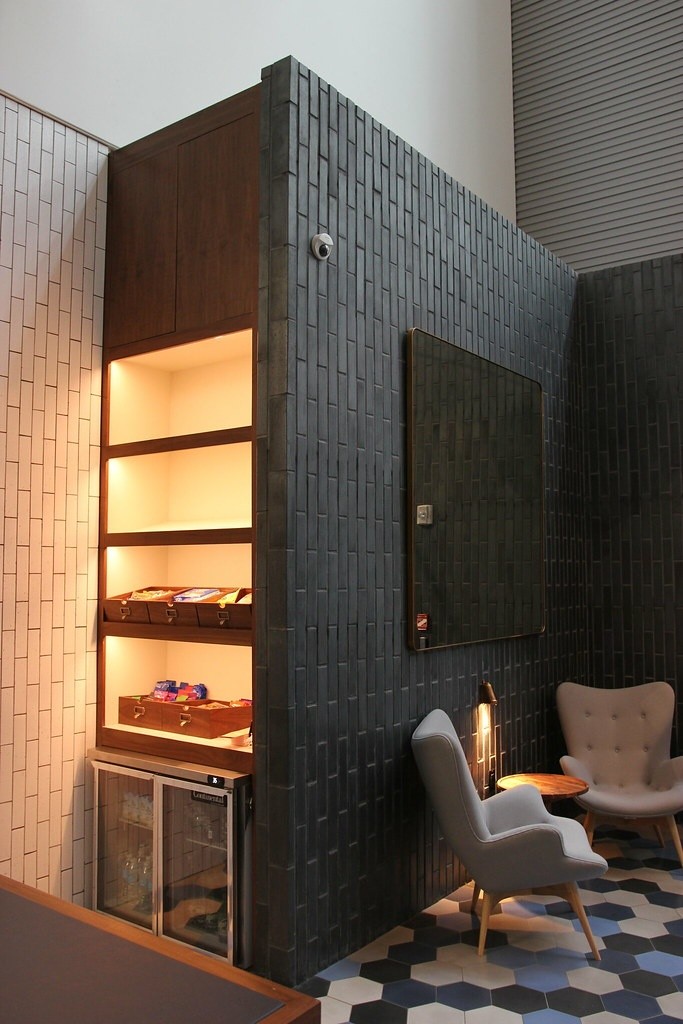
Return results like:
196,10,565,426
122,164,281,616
497,772,589,818
0,873,324,1024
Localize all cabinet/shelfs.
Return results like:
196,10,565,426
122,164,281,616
87,746,254,972
80,80,262,777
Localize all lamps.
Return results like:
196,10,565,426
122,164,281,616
478,680,498,706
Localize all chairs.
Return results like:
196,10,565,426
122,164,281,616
553,681,683,868
410,709,608,961
